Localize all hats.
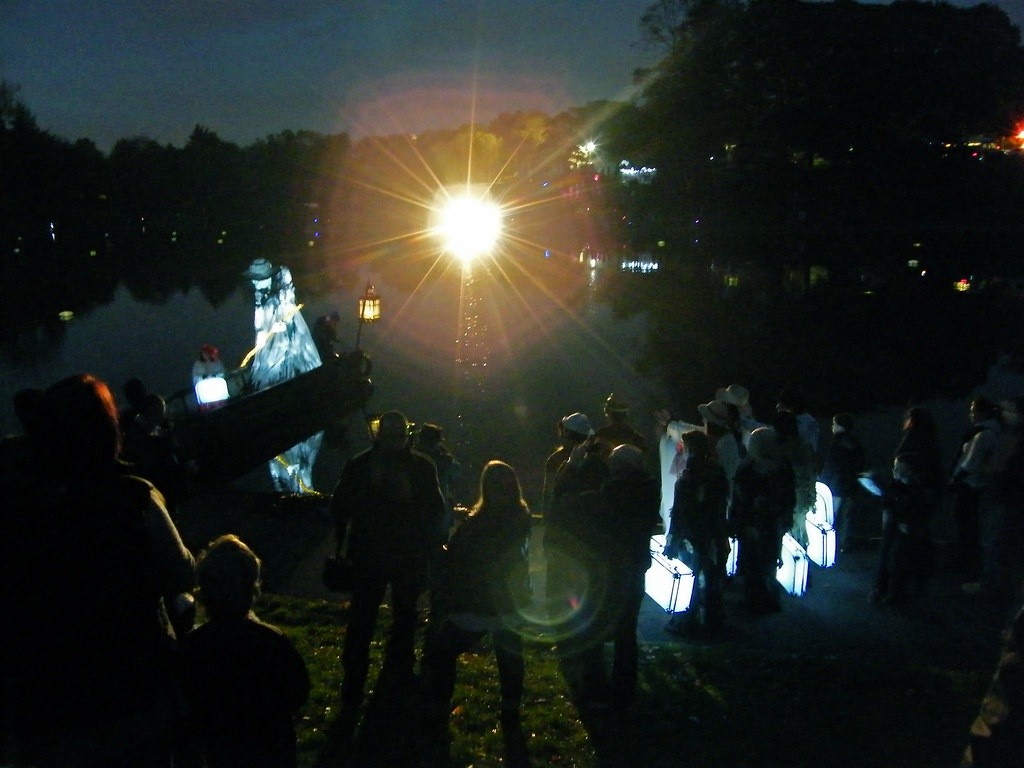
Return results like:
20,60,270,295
605,390,629,413
698,384,753,427
417,421,445,443
748,426,791,462
562,413,594,436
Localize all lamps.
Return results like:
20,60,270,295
359,281,382,327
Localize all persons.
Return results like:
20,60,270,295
664,430,728,637
590,390,647,467
728,427,794,620
698,400,747,507
553,438,662,705
1,387,46,486
717,384,757,435
421,459,536,725
410,420,458,528
762,385,1021,615
543,413,598,604
191,258,340,406
328,410,450,704
1,375,195,768
168,534,308,767
119,377,178,476
